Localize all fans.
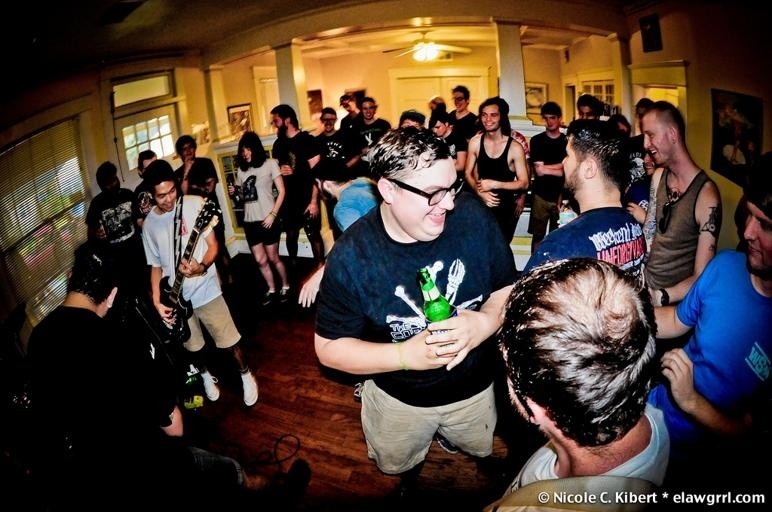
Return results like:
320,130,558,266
383,28,473,63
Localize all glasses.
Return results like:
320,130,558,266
385,174,465,206
659,201,672,233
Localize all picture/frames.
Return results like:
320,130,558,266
523,80,548,116
224,102,256,142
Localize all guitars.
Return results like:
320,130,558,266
151,198,216,343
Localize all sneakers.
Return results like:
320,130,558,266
279,287,291,303
200,369,222,402
240,367,259,408
260,290,276,306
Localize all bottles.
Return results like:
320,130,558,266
416,267,450,320
558,200,574,225
228,180,241,206
